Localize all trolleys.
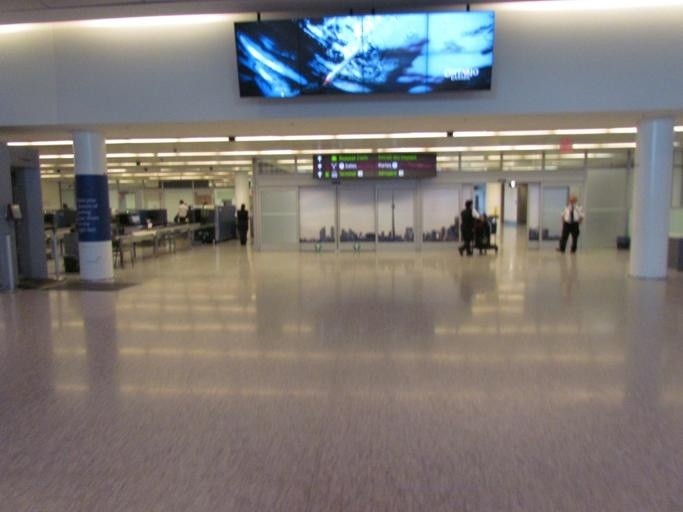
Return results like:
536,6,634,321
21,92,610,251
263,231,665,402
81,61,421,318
476,214,498,255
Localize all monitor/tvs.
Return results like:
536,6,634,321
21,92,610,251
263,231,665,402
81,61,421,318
8,203,24,220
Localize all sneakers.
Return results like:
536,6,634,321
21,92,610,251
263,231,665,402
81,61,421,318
458,247,472,255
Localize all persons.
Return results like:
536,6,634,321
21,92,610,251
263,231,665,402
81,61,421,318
177,199,189,224
237,204,248,245
458,200,482,255
555,195,584,253
